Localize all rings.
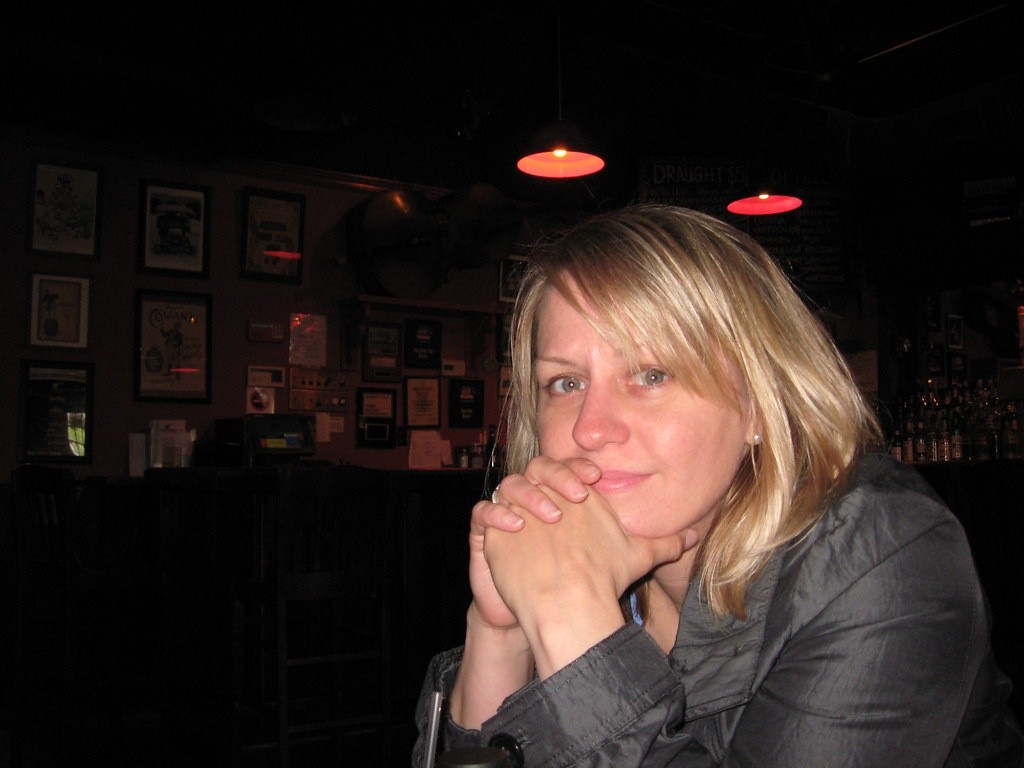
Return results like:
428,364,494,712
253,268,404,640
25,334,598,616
492,485,500,504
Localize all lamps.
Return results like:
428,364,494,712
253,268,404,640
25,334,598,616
518,0,606,181
726,1,805,217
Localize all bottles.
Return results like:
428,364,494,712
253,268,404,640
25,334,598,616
484,426,501,467
890,379,1019,463
456,449,468,467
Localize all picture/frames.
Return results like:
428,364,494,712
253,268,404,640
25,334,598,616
29,276,91,347
133,287,215,405
353,250,537,452
926,350,945,375
237,180,308,284
140,176,213,279
928,294,941,331
28,160,103,258
945,311,965,351
16,355,99,465
946,352,968,387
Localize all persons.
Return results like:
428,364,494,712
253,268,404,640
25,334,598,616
414,202,1024,768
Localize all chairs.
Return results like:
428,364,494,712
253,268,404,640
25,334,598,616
223,571,395,767
